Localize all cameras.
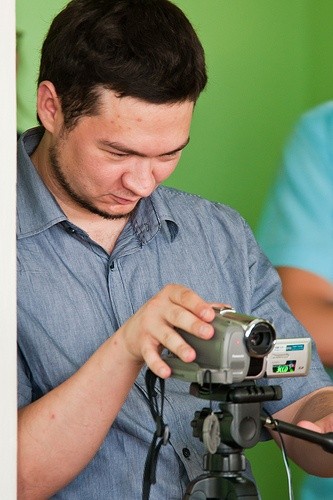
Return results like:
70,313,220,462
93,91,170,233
161,306,313,387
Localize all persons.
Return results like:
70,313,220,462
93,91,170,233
17,0,333,500
254,93,333,500
